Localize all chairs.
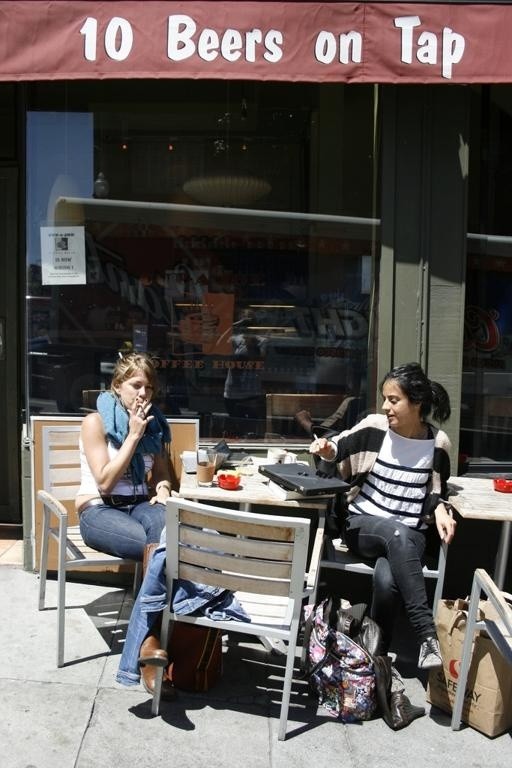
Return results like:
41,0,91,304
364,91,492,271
263,392,349,441
34,424,511,741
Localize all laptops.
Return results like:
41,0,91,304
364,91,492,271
257,463,351,497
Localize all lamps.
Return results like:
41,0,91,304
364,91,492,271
44,78,110,227
181,81,273,210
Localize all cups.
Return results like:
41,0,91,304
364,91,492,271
264,433,285,443
286,452,297,464
266,448,292,464
195,450,216,487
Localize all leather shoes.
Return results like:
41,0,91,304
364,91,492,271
138,635,175,698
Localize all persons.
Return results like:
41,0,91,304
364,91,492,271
76,352,180,699
216,308,277,438
295,365,366,439
309,361,458,691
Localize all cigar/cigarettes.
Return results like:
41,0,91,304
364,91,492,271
313,434,320,445
137,403,142,406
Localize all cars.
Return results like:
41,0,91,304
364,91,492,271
22,291,374,433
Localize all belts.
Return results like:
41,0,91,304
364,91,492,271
79,494,147,513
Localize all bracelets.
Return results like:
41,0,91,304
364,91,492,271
158,484,170,492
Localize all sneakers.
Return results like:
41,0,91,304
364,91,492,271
418,636,443,669
390,665,406,694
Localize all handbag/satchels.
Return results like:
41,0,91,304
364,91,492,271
172,623,222,692
303,591,376,723
425,597,512,737
